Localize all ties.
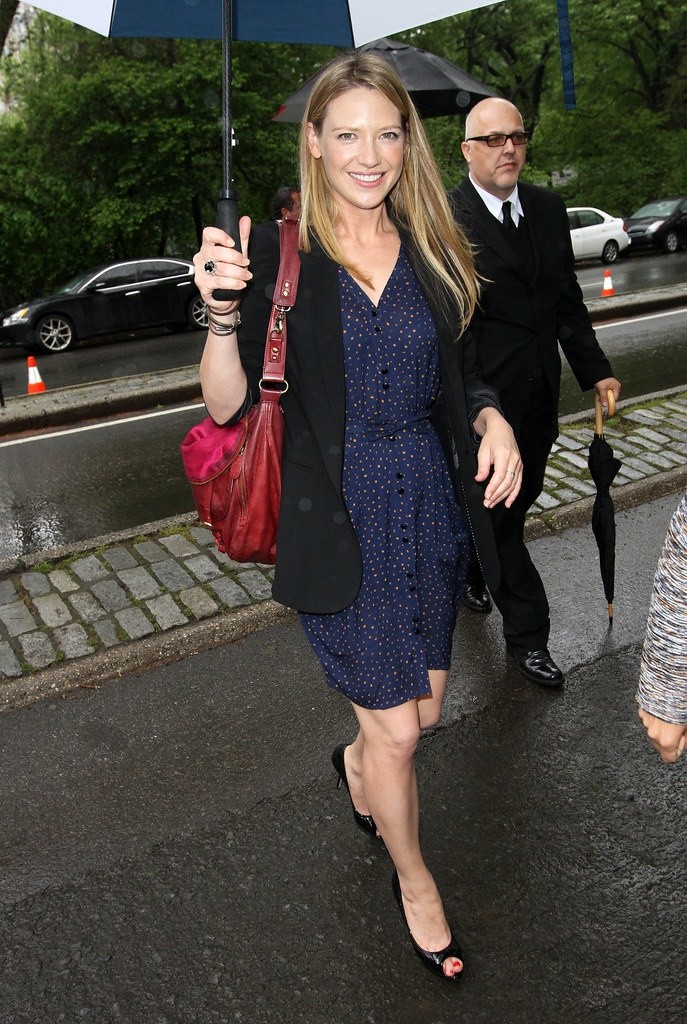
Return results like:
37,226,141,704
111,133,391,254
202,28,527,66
502,201,516,230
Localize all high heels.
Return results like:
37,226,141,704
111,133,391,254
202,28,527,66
331,743,382,839
392,867,466,983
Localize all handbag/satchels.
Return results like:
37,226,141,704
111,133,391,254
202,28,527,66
181,218,299,565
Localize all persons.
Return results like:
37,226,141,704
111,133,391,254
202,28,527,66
444,96,621,686
272,187,299,219
636,487,687,764
193,54,523,981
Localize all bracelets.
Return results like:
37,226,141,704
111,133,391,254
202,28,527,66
206,302,242,336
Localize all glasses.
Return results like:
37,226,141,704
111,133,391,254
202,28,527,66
466,132,530,147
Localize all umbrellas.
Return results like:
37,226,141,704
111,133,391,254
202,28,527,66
270,36,502,124
587,389,623,623
19,0,510,301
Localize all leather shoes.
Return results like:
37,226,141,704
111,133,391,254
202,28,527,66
462,583,493,613
513,645,565,684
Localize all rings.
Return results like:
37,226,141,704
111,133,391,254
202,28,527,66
205,259,218,277
507,470,514,475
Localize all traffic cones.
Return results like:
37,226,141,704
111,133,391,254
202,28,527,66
26,356,46,394
601,269,615,297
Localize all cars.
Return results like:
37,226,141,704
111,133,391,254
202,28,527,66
567,207,632,265
624,195,687,254
0,256,216,355
